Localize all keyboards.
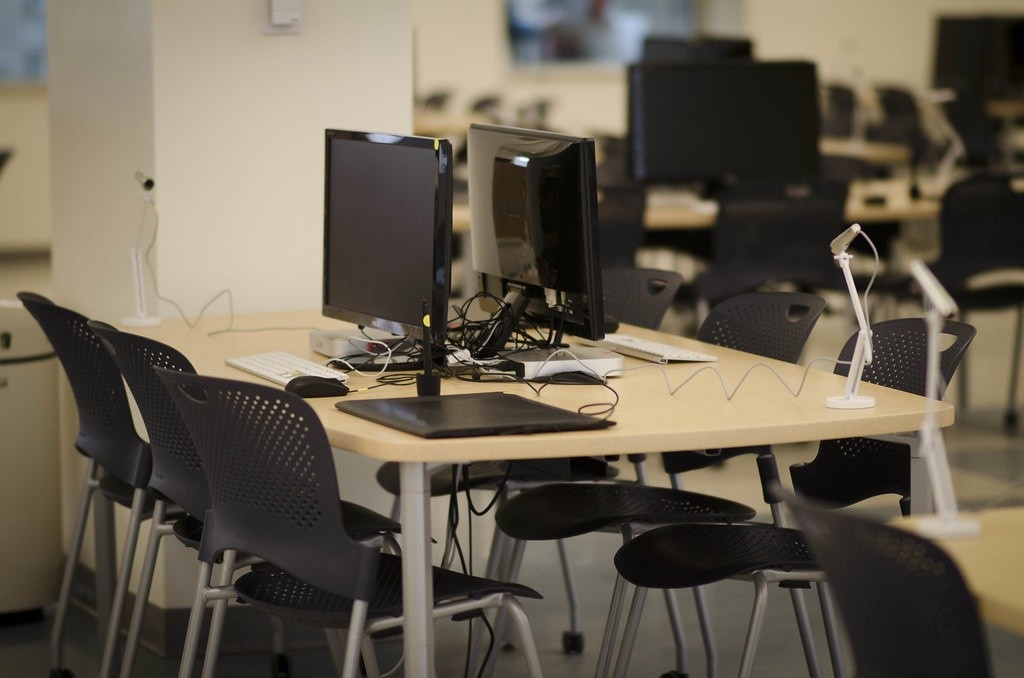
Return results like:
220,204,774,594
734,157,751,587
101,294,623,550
576,334,719,363
226,350,349,387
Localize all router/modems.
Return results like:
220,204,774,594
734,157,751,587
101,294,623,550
309,327,415,359
494,332,626,381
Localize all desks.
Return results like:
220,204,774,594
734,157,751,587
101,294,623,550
86,319,956,678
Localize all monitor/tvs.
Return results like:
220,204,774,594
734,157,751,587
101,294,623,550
631,60,824,205
322,129,458,379
641,35,752,61
934,16,1024,103
463,123,606,360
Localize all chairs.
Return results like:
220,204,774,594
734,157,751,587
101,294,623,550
17,271,976,678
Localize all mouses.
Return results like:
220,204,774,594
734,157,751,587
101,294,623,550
604,313,619,334
285,375,349,399
